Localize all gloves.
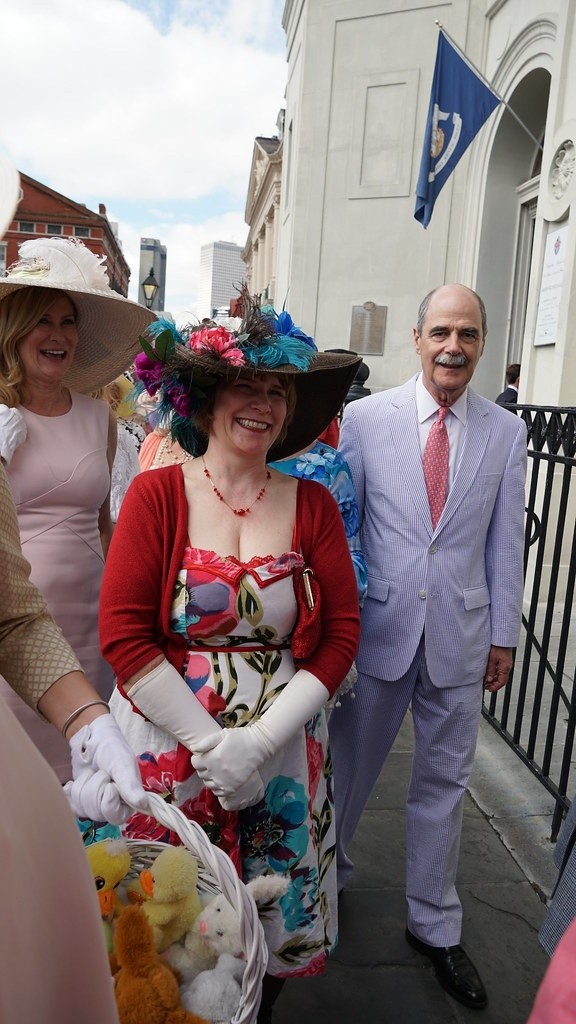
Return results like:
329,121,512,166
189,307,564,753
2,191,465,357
127,659,265,812
62,713,148,825
190,669,331,798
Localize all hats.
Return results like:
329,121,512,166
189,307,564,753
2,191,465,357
0,238,159,395
136,300,363,463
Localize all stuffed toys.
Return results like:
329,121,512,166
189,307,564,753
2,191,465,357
84,838,291,1024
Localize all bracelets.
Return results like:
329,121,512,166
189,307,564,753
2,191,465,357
61,700,111,740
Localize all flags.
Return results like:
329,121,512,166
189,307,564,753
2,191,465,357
414,31,501,229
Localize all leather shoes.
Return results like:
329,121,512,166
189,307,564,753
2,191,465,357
406,927,489,1012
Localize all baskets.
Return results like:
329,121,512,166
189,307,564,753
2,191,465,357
60,781,268,1024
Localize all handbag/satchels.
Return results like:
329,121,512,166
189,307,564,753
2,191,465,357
293,568,321,660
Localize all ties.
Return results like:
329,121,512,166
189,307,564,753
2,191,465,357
424,407,449,531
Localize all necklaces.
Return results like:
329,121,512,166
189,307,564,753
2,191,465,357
201,455,271,516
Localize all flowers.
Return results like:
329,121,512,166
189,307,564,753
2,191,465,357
132,303,318,418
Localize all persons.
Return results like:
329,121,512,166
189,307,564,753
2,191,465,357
0,236,168,1024
96,279,366,1024
496,364,521,414
526,798,576,1024
328,283,528,1009
